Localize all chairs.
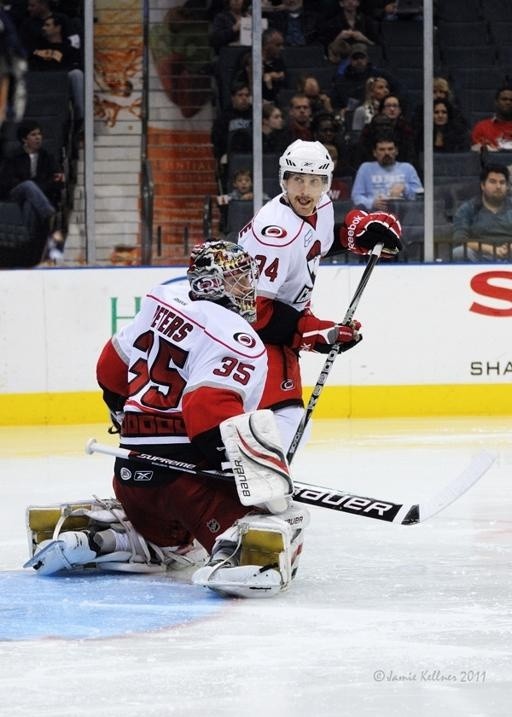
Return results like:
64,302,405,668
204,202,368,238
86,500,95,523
1,0,87,269
214,0,511,263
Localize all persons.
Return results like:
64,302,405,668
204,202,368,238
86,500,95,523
30,239,311,597
0,0,511,268
239,139,337,480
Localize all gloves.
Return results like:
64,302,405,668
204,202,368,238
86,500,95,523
290,308,362,353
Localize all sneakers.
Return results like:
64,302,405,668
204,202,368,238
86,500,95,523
34,532,96,574
192,564,281,597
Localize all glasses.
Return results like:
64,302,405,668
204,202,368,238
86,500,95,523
352,52,365,59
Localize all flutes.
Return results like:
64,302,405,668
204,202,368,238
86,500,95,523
84,438,494,525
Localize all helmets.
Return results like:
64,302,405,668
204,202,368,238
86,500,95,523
279,139,334,194
187,239,258,315
